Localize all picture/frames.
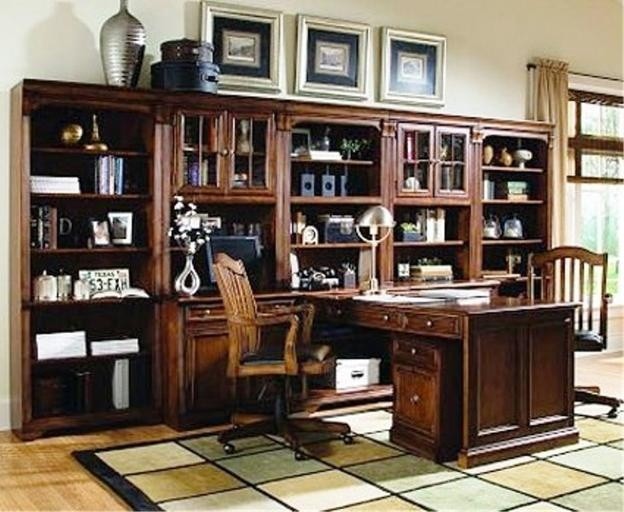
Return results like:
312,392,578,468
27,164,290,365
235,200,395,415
198,0,448,108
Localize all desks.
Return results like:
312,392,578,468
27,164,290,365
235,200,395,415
301,291,580,468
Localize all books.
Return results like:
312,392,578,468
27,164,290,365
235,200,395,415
30,175,81,192
203,214,269,246
406,133,416,161
481,171,495,199
32,331,139,412
409,264,454,280
501,179,532,199
93,154,124,195
183,152,209,186
29,204,58,248
413,206,448,242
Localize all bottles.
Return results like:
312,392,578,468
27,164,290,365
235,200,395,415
398,249,410,282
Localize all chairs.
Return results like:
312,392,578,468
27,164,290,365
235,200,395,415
211,251,354,461
526,245,623,421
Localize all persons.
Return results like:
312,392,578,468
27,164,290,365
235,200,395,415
93,222,107,242
113,218,130,238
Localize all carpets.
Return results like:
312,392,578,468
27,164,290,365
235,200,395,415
66,395,623,512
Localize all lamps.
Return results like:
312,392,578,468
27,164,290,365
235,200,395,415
351,202,396,297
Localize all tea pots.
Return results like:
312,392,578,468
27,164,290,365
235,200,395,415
482,212,524,239
